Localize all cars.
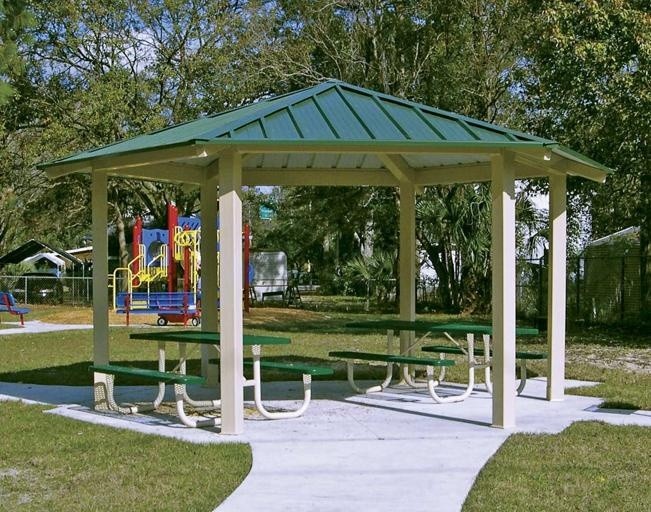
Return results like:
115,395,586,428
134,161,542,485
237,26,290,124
12,273,64,306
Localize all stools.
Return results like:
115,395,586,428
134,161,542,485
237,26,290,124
244,286,257,305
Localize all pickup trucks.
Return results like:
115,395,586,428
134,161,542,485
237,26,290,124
291,279,323,295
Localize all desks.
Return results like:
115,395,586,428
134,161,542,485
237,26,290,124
345,320,539,403
128,330,291,426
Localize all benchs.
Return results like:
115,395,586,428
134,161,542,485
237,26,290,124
327,346,547,403
261,291,284,301
87,359,334,426
0,292,30,325
